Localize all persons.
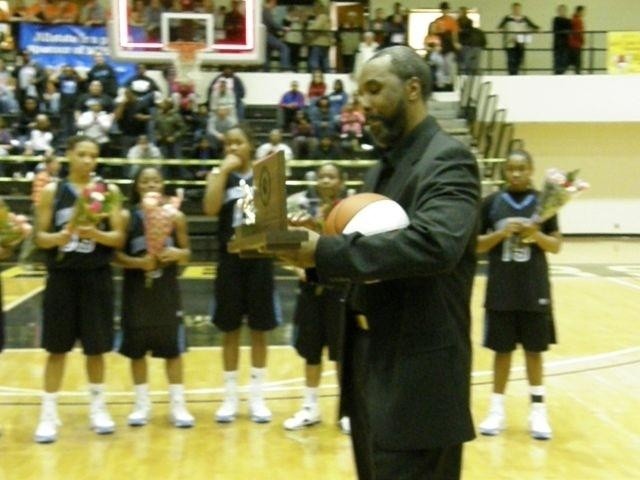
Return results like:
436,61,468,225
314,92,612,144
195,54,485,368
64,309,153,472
570,6,584,75
497,3,539,76
553,4,573,75
283,163,352,432
0,201,20,358
201,125,281,425
32,136,128,443
474,151,562,440
0,0,488,269
111,166,196,427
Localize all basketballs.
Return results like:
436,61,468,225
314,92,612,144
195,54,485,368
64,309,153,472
323,190,409,239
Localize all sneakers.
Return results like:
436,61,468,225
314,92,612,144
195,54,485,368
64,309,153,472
247,396,271,422
34,411,61,442
283,404,321,430
477,408,507,436
126,393,153,425
168,398,195,427
529,403,552,440
214,396,240,421
87,403,116,434
